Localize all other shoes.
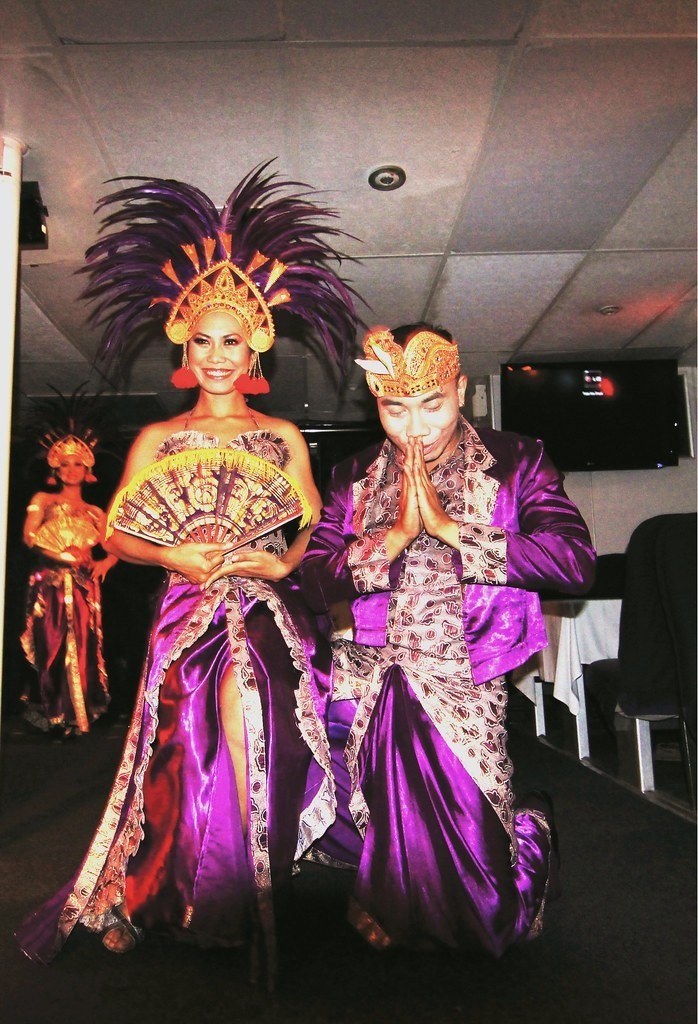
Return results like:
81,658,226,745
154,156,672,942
347,900,397,952
48,718,84,739
103,926,140,958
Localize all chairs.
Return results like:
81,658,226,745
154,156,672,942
593,552,626,597
583,512,696,793
652,517,696,812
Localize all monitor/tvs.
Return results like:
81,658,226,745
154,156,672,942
500,357,680,472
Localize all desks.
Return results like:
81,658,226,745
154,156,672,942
510,598,623,767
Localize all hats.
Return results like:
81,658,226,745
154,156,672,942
72,156,378,392
29,382,126,485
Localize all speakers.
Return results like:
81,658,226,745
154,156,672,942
19,182,51,251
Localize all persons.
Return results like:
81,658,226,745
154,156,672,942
22,433,119,735
12,305,595,1024
